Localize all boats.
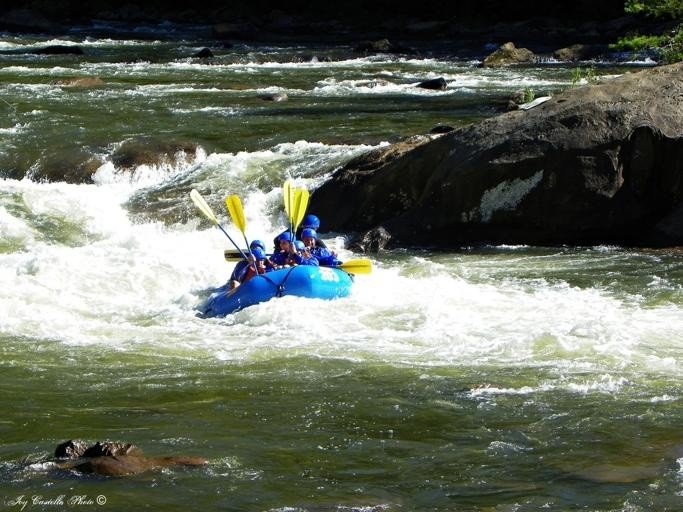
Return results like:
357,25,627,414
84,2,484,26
203,265,354,318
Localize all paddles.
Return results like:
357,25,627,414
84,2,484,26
224,249,274,261
226,194,259,275
321,260,372,274
283,182,294,242
292,189,309,241
190,189,251,266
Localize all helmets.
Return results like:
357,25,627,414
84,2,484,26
278,215,320,251
250,240,266,260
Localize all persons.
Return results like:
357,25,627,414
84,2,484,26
227,214,342,287
